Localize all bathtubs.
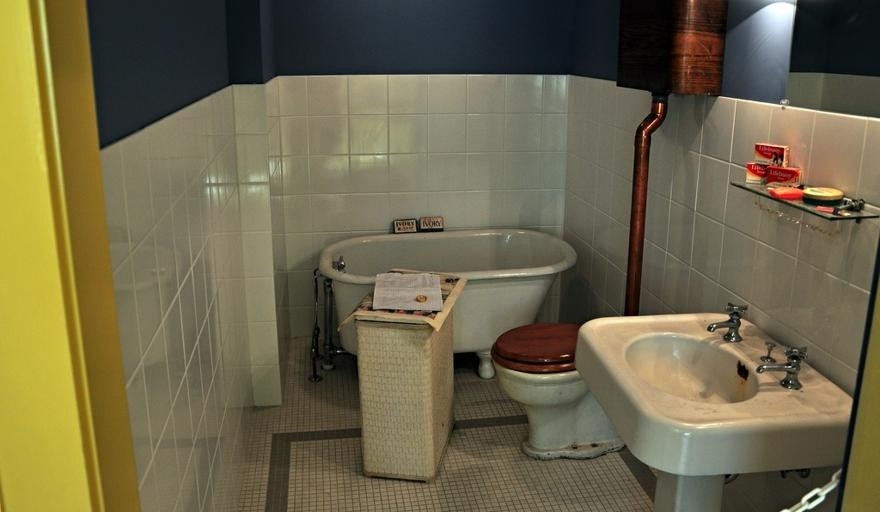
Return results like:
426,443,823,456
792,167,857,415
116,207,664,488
319,228,577,379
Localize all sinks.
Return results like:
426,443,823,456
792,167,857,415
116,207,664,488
574,311,855,476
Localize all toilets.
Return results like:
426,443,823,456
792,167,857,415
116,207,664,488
491,322,626,461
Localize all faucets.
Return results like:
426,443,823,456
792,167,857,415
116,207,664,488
757,347,808,390
707,302,748,341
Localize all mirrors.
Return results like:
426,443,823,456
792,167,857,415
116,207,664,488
785,0,880,118
